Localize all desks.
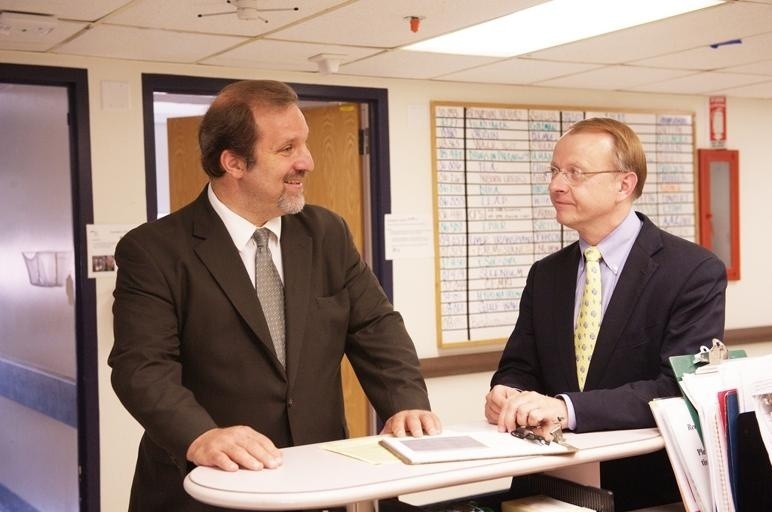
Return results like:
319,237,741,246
182,420,667,512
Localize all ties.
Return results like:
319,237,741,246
573,245,605,392
251,228,288,369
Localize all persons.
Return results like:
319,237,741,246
103,77,446,511
480,116,730,512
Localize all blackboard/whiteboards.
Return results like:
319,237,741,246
430,101,699,347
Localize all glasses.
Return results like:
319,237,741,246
540,164,625,184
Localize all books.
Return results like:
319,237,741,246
647,354,771,512
